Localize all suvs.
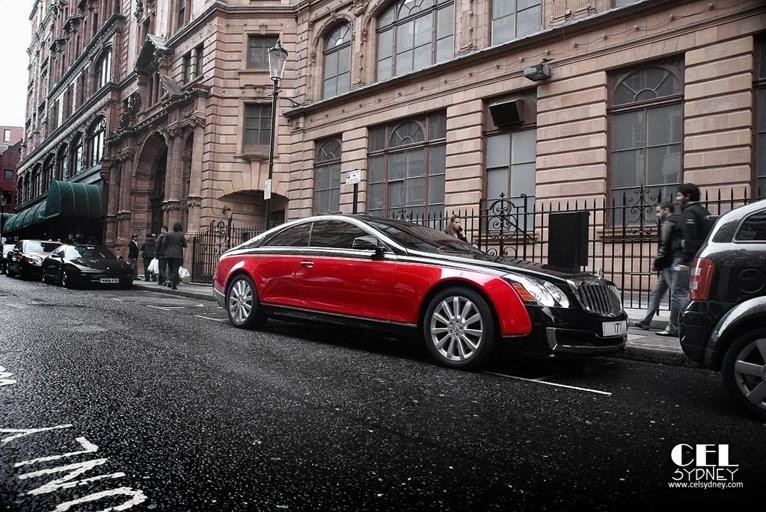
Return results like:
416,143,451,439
675,197,765,421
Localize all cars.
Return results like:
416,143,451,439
210,209,629,373
0,244,15,274
6,238,65,279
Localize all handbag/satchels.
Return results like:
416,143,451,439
147,257,158,276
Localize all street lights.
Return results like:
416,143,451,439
260,37,288,231
0,194,7,239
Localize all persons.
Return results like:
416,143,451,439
162,222,188,290
152,234,157,240
127,234,141,280
442,214,467,242
655,183,710,337
141,233,157,282
630,201,680,331
156,226,168,284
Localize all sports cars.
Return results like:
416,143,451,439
39,243,134,293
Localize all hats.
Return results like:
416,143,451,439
131,234,138,238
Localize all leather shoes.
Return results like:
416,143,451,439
655,330,678,336
631,321,649,331
133,276,178,290
